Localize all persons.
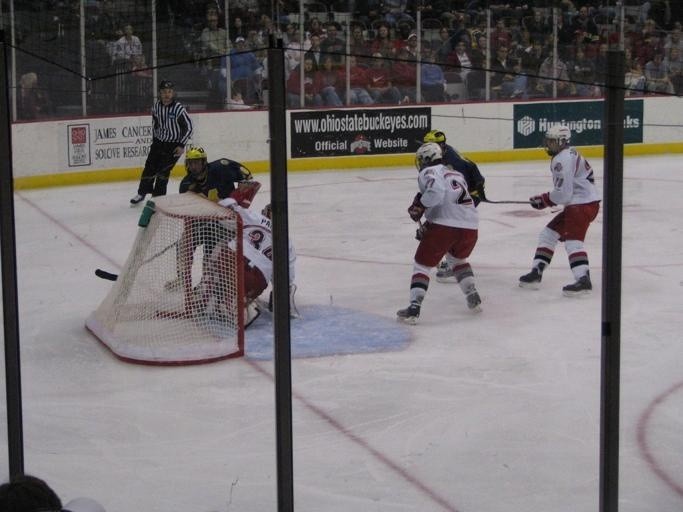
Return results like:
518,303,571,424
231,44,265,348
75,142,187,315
397,141,487,319
130,77,193,208
1,0,682,121
190,178,296,333
518,124,602,293
414,129,486,277
166,143,252,293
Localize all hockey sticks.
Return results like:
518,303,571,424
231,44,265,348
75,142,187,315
476,181,534,204
95,239,176,281
414,211,449,272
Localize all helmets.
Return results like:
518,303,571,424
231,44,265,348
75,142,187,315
544,125,570,156
261,204,272,220
159,79,175,93
185,146,206,173
415,142,442,172
423,129,445,143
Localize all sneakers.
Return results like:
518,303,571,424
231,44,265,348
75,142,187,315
562,270,591,290
244,301,260,329
164,279,191,292
519,262,544,282
396,296,423,318
130,194,144,203
464,283,480,309
436,260,453,277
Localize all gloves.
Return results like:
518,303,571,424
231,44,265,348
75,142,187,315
529,191,556,210
415,220,428,241
407,202,424,222
230,180,260,208
